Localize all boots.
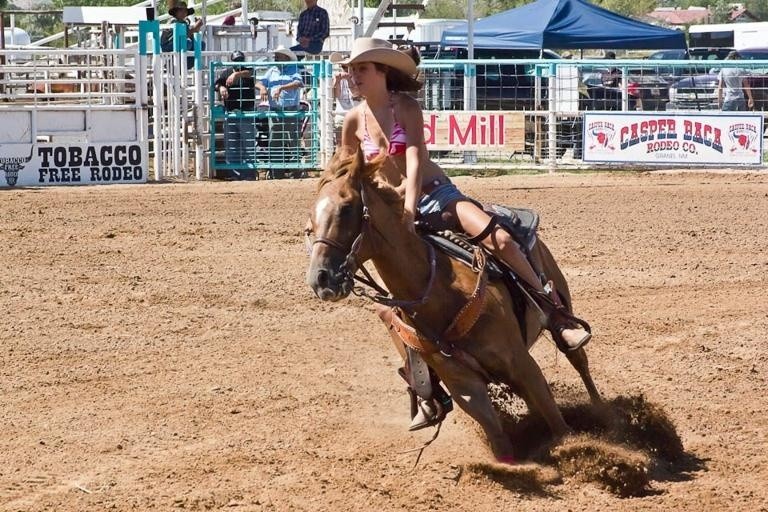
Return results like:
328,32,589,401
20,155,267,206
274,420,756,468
399,358,454,432
530,279,592,354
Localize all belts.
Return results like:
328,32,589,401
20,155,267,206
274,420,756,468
419,177,450,201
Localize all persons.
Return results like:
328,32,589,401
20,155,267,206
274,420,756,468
160,0,206,68
599,52,623,88
289,0,329,94
333,58,362,146
340,36,591,431
215,50,256,180
717,51,755,112
254,45,305,179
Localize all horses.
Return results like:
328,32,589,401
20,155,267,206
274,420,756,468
306,141,607,467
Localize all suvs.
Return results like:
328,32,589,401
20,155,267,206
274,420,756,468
579,48,768,134
431,46,638,109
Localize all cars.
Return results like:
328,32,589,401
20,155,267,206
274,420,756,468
25,69,167,102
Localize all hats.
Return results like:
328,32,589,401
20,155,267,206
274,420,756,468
231,50,244,61
329,36,417,75
167,0,194,18
267,45,297,62
